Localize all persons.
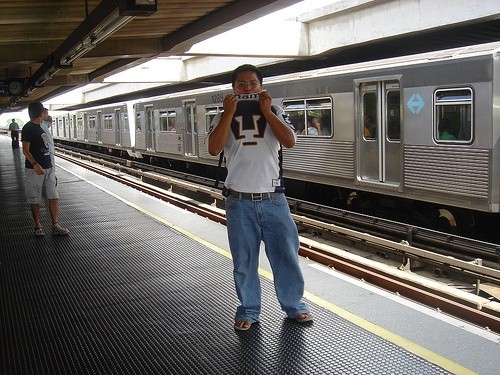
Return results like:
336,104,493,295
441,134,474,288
363,112,379,141
208,65,315,331
294,114,332,136
8,118,22,151
438,121,472,141
23,100,71,237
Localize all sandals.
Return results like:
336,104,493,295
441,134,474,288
51,222,70,235
33,223,45,235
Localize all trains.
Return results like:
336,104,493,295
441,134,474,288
50,40,500,228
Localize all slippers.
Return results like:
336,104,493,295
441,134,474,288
296,313,314,322
234,320,251,330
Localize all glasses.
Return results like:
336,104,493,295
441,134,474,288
235,82,260,88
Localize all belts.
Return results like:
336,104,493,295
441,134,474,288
228,188,275,201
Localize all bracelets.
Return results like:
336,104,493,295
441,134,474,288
32,162,37,166
